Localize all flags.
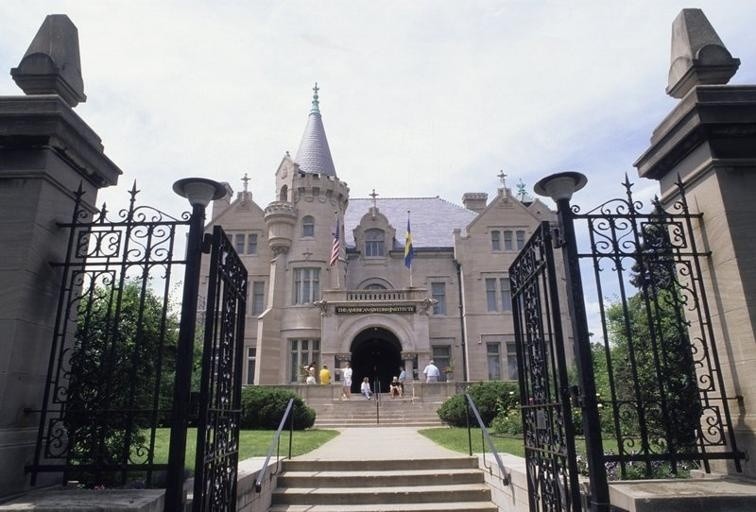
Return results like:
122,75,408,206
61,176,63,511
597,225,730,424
330,217,339,267
404,213,414,268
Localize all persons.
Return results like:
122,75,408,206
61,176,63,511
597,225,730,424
361,377,373,400
304,361,317,384
319,365,331,384
423,360,440,383
390,376,403,399
335,362,353,399
399,366,407,393
